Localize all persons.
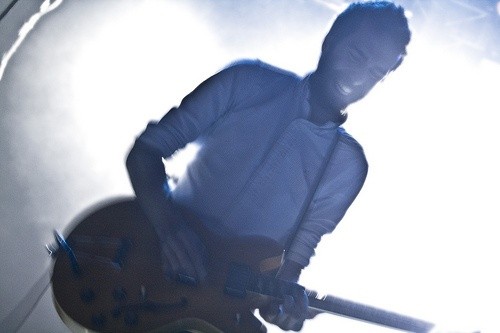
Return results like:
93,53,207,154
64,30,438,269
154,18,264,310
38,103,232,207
126,0,412,333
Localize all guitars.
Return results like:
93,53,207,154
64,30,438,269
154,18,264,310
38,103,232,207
49,194,433,333
